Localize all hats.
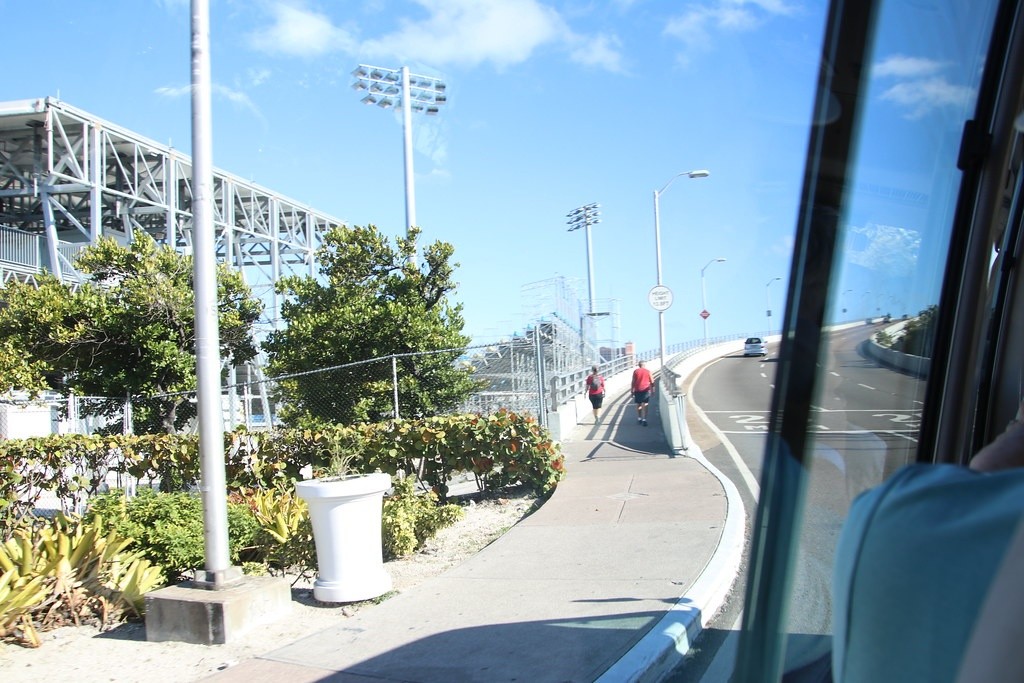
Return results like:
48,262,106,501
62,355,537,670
639,360,647,365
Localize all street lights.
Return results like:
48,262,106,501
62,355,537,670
765,277,782,336
565,201,602,310
701,257,724,346
653,168,710,367
352,63,448,268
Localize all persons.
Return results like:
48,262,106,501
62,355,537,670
825,397,1024,683
584,364,607,426
631,360,656,426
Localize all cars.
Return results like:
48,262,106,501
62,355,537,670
742,335,769,356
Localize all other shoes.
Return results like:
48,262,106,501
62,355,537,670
595,419,601,425
637,417,648,426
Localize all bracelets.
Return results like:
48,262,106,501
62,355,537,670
1007,418,1023,427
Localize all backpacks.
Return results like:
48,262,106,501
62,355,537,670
590,374,601,391
